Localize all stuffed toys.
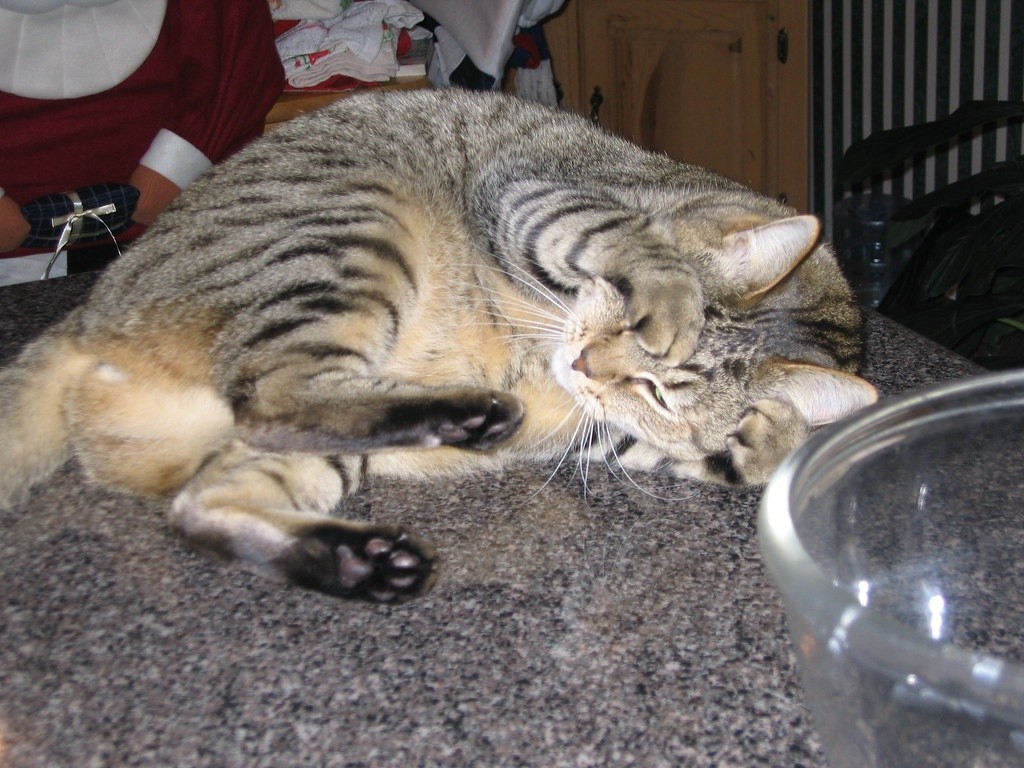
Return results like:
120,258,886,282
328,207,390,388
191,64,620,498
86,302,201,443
0,0,286,286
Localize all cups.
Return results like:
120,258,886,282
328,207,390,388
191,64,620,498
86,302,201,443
756,367,1024,768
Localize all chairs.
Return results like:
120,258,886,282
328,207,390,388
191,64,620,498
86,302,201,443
833,99,1024,371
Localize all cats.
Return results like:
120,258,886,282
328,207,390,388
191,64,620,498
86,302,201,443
0,87,879,604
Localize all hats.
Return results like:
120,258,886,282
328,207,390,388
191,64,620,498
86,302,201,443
0,0,167,100
